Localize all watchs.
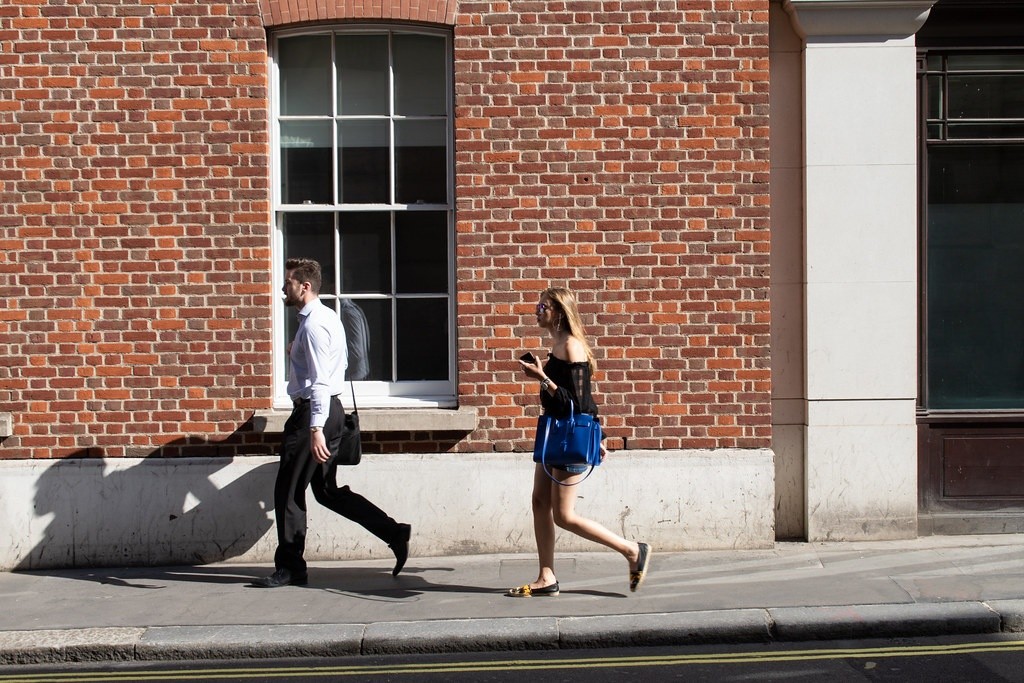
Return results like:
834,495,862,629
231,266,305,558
310,427,323,432
540,377,551,390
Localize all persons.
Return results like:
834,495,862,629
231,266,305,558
340,299,369,381
509,287,653,598
250,257,411,587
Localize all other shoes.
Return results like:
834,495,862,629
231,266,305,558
629,542,653,593
508,579,559,598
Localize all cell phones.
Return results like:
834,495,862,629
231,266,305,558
520,352,535,364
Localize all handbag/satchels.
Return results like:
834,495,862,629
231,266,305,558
533,399,601,486
337,411,363,465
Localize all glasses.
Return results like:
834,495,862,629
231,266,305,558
535,304,553,311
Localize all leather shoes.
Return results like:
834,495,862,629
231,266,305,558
388,521,411,577
253,570,307,587
